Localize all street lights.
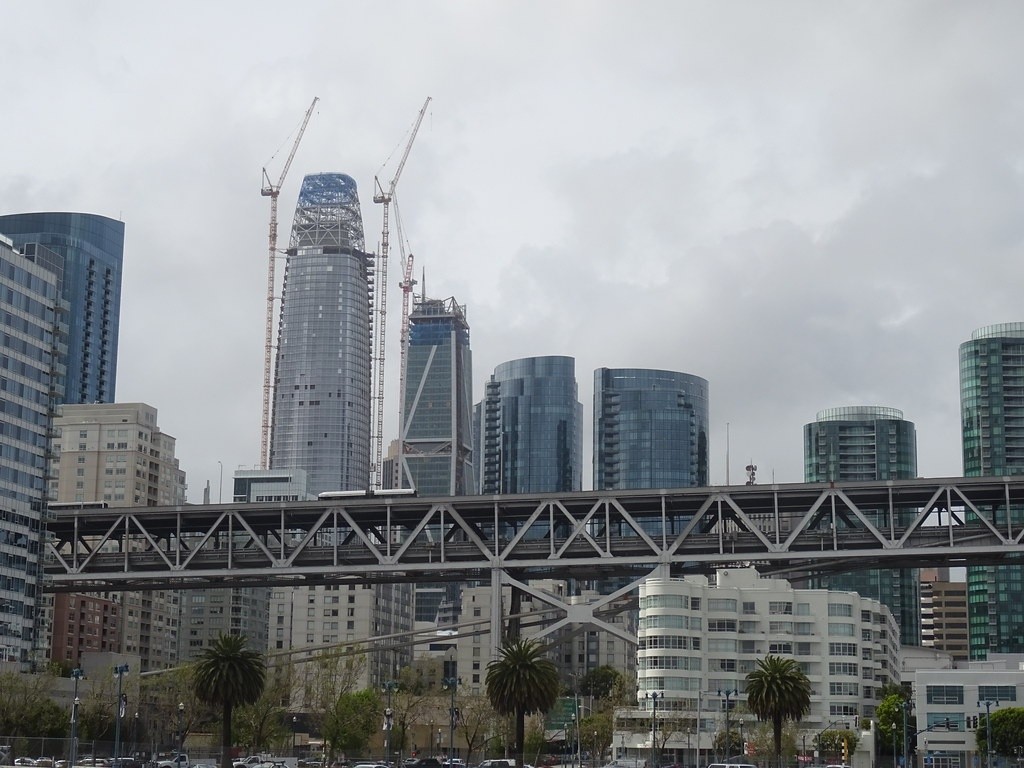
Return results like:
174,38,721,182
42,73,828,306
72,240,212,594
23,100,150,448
177,701,185,768
380,680,400,766
569,672,582,768
113,663,129,768
440,676,463,768
892,723,897,768
739,717,744,756
802,735,806,768
975,697,999,768
69,668,85,768
717,687,738,763
292,715,298,757
645,689,664,768
133,712,139,762
687,727,691,768
218,461,222,505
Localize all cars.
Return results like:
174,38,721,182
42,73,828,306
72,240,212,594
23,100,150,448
12,749,648,768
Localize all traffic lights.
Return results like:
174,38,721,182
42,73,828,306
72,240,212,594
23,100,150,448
973,716,978,728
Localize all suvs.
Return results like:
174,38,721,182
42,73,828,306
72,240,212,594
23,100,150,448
707,763,757,768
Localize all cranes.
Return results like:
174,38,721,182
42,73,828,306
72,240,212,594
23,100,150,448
259,96,320,471
369,96,434,487
